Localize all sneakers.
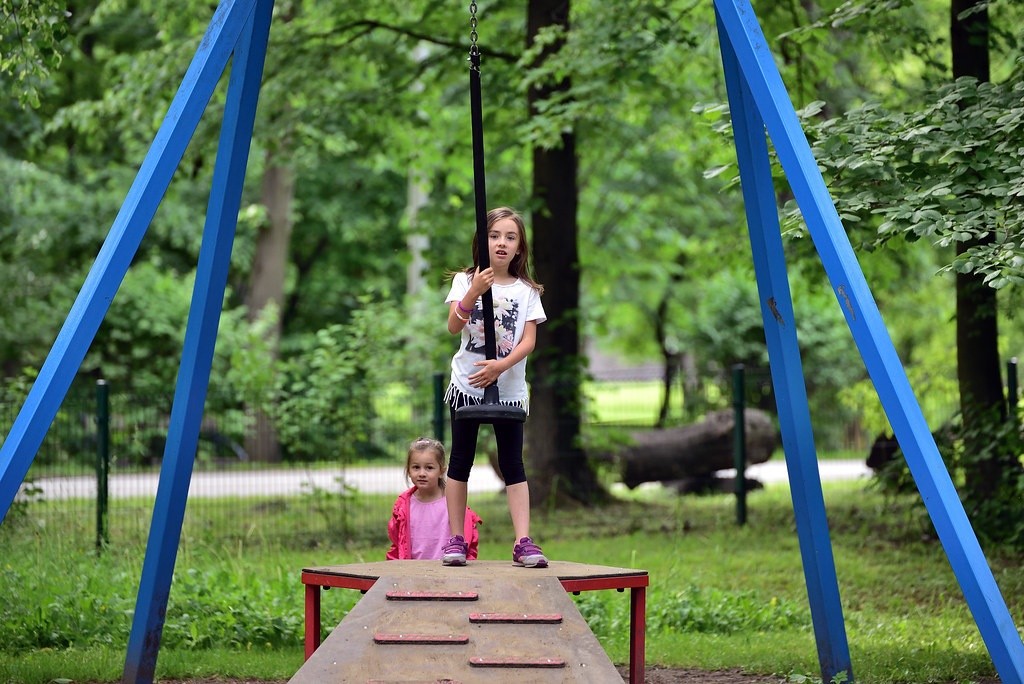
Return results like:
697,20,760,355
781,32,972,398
511,536,549,568
441,535,468,566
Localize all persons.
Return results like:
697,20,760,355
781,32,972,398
387,437,482,560
443,208,548,567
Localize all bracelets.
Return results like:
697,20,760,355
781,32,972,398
454,306,471,323
459,302,474,313
